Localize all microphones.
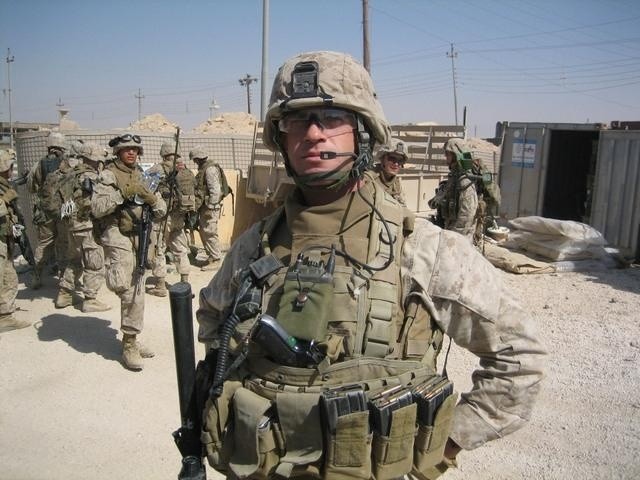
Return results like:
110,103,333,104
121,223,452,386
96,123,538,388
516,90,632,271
320,150,358,160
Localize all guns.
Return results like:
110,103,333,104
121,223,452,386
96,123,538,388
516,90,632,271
9,199,41,280
251,312,326,368
171,282,207,480
137,204,153,295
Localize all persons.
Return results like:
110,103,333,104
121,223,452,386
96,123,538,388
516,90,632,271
89,133,157,374
370,134,410,213
427,135,503,256
196,47,548,480
1,129,230,333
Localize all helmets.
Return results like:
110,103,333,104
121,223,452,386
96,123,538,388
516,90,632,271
445,138,467,154
0,148,17,173
262,50,408,161
46,132,208,161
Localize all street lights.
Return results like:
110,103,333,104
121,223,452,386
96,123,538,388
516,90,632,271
4,46,14,151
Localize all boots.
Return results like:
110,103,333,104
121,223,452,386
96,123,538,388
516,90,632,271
0,263,111,332
146,274,195,299
201,255,222,271
121,334,155,370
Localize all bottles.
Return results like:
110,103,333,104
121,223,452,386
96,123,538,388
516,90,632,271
134,171,161,205
